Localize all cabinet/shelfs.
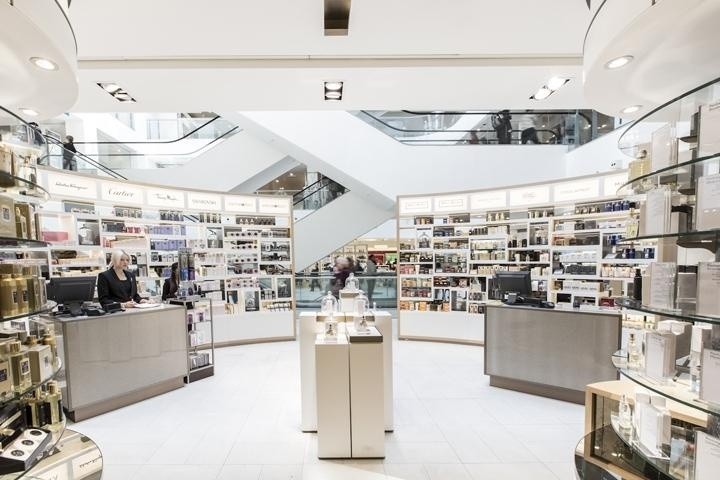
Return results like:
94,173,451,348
398,168,646,345
36,165,294,348
574,79,718,480
166,296,214,385
0,105,103,480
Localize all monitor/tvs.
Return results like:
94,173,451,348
496,271,532,305
50,276,96,317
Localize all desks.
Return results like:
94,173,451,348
39,301,188,422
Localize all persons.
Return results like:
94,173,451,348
163,262,178,300
62,135,76,170
98,247,154,313
467,108,542,143
311,253,379,301
